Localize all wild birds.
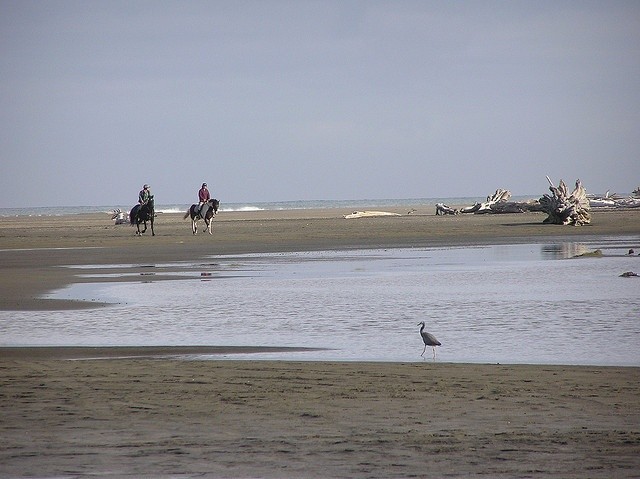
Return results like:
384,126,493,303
417,321,443,358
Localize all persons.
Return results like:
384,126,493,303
137,182,157,221
198,182,210,219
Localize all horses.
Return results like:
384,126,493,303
184,199,222,236
130,195,156,237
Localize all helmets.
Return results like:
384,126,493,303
144,184,149,188
203,183,207,186
